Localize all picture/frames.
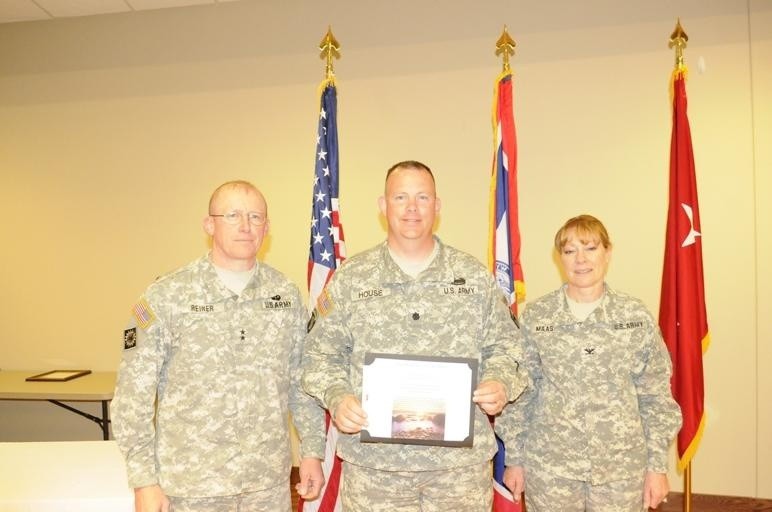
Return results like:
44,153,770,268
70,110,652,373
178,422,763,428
362,353,478,452
27,367,94,384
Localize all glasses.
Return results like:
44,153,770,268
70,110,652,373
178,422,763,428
208,210,268,227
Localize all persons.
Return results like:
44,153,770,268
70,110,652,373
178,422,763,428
498,216,684,512
112,179,327,511
298,161,530,511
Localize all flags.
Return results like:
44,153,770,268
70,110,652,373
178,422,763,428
485,69,532,512
658,65,710,476
298,67,350,511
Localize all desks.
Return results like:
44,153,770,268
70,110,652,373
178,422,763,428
1,367,112,441
